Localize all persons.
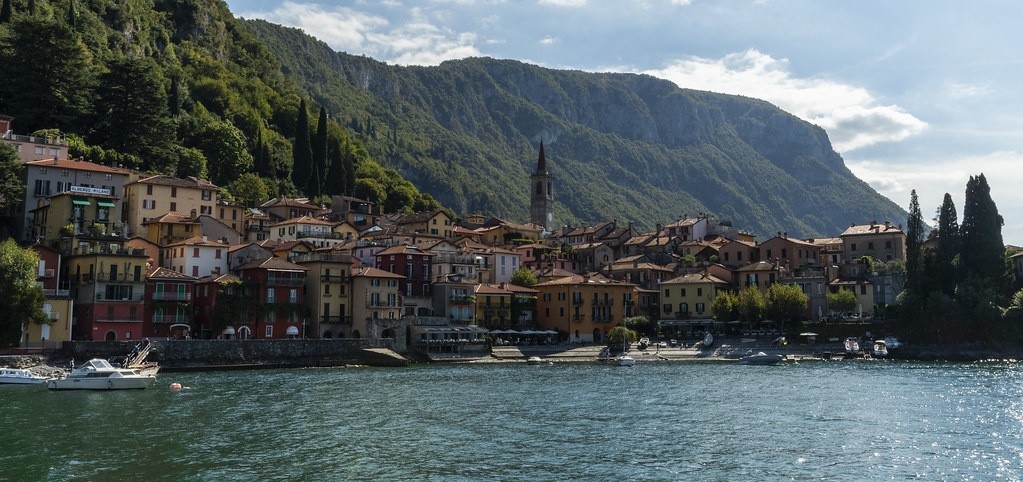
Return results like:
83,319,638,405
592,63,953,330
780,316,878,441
69,358,74,373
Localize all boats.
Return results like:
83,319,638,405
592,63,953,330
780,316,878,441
660,341,667,348
617,356,636,366
48,358,163,390
670,338,677,347
703,331,713,347
637,336,650,349
0,368,50,384
844,336,860,354
885,334,899,349
874,340,889,357
740,352,783,364
